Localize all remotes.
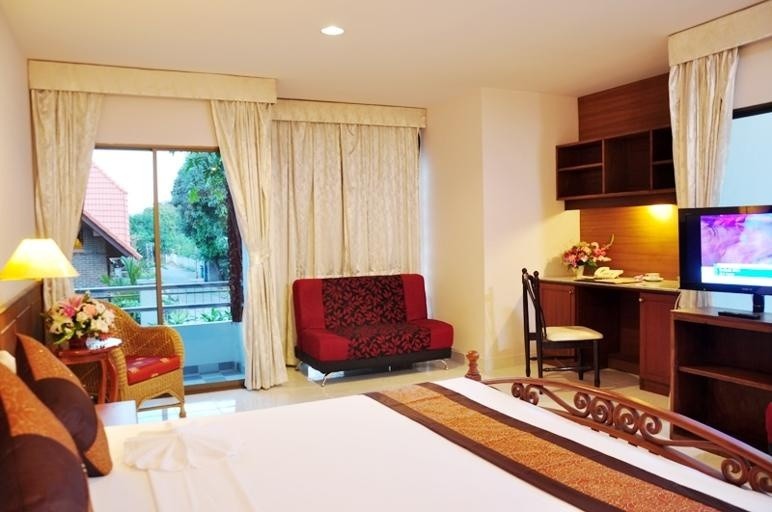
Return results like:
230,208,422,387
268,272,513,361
717,309,759,319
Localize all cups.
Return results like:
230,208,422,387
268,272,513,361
646,273,659,280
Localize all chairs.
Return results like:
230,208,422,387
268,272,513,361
522,268,605,387
83,300,186,417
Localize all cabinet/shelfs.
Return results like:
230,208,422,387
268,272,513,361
668,308,772,455
555,125,675,199
539,280,681,396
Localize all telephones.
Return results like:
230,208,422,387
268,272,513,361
593,267,623,279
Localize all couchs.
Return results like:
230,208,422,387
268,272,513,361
293,274,454,386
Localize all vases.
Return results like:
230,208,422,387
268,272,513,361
576,265,584,278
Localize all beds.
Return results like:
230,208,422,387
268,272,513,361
85,351,772,511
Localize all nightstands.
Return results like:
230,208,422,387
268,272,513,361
94,398,138,426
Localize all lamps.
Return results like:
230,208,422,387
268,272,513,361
0,239,79,343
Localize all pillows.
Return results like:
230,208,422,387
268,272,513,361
0,348,16,371
1,364,90,512
13,332,111,475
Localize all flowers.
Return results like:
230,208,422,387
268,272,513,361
39,289,116,341
562,234,614,274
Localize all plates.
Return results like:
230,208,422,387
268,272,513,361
644,277,663,282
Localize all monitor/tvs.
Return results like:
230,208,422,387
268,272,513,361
677,208,772,311
